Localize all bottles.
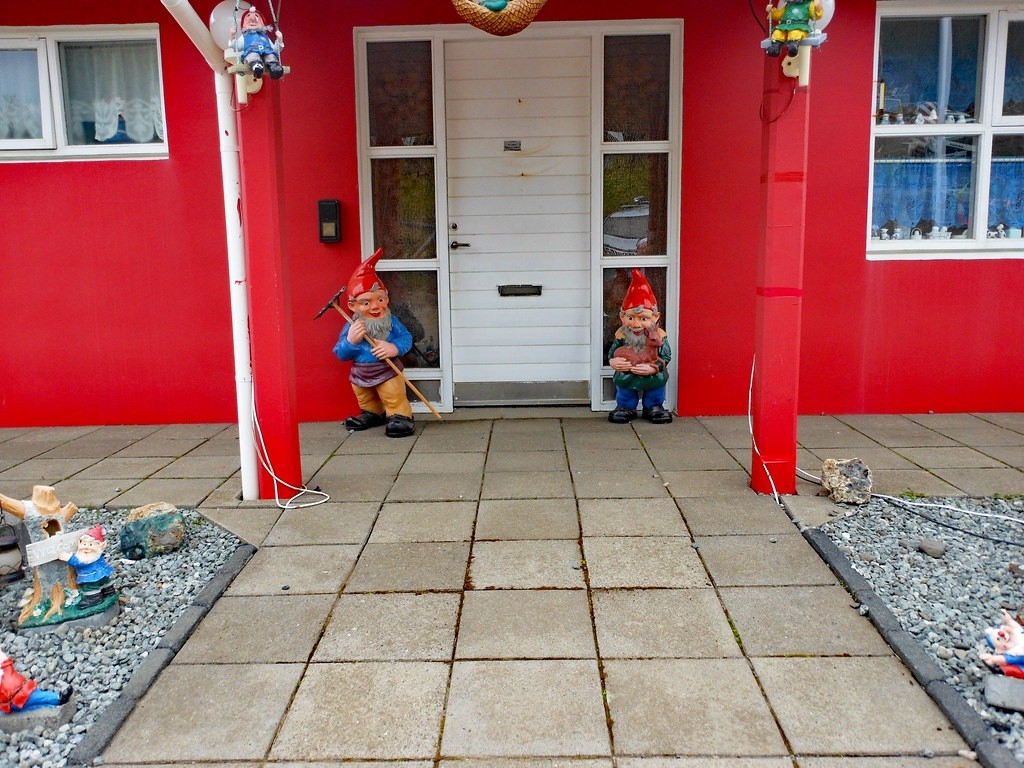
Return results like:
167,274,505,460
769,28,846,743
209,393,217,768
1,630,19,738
892,228,903,240
880,114,891,125
895,114,905,125
880,228,890,240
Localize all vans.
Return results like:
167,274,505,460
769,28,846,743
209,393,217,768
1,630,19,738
603,203,649,255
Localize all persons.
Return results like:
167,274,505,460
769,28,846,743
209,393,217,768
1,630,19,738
334,247,416,437
607,269,673,423
227,6,285,78
58,524,117,610
